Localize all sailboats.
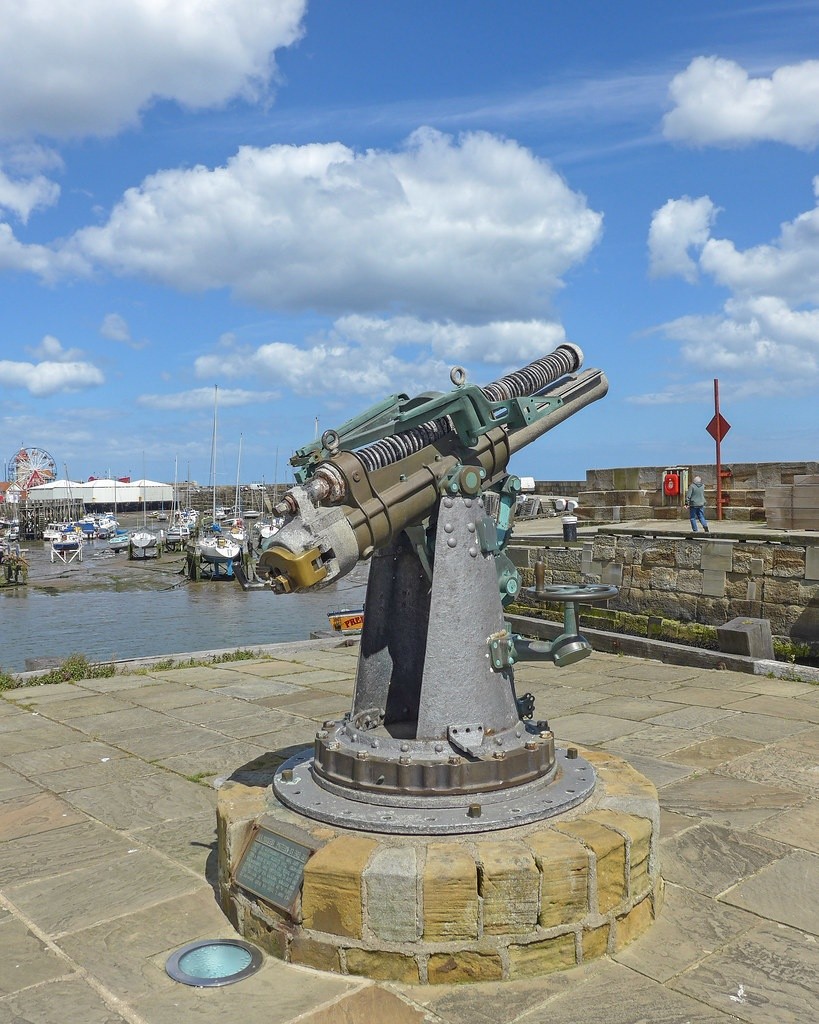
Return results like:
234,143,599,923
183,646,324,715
0,456,20,541
52,384,277,564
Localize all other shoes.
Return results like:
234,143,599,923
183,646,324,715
703,526,709,532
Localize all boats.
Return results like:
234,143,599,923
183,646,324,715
327,604,364,630
43,522,65,540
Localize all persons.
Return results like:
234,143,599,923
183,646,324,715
57,535,68,557
686,476,709,532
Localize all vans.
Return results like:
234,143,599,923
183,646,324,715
518,477,536,496
250,484,266,491
234,486,248,492
